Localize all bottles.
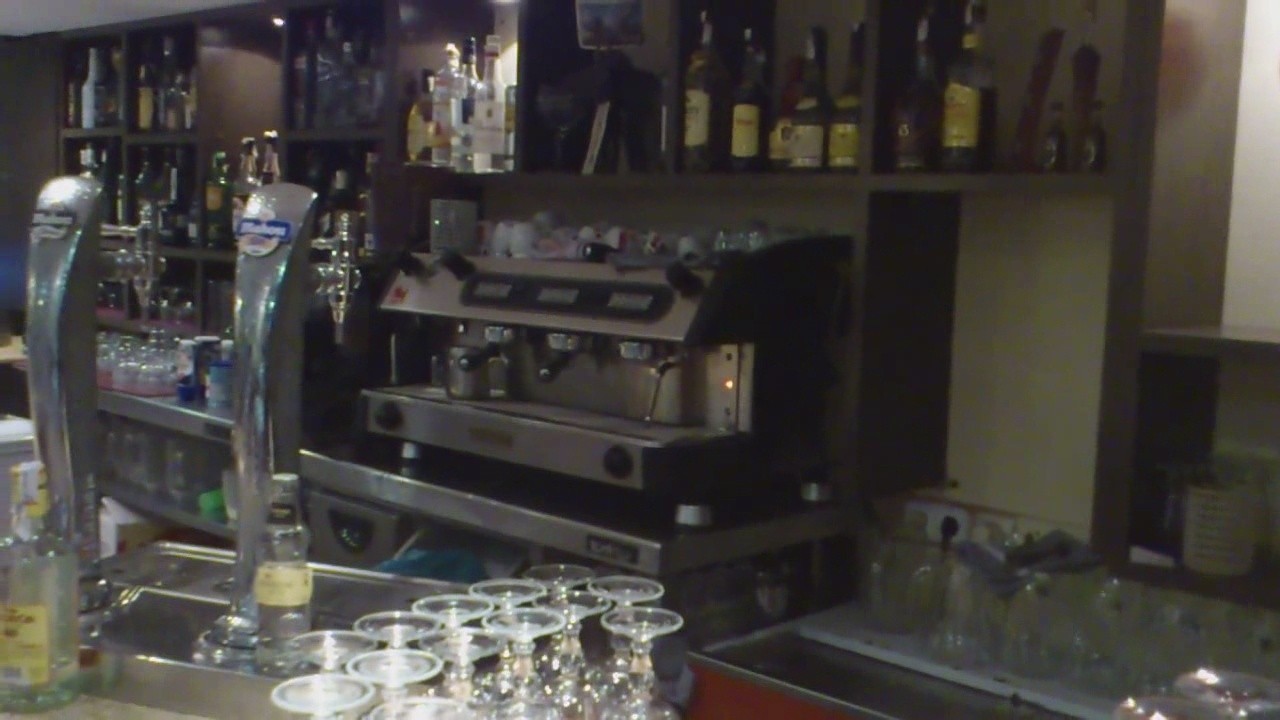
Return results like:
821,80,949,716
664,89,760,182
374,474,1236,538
532,1,1104,175
60,24,281,253
0,462,78,713
290,7,518,265
255,474,313,630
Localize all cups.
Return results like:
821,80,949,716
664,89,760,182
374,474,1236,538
427,198,478,255
90,210,194,394
445,344,484,397
861,517,1280,720
194,563,697,720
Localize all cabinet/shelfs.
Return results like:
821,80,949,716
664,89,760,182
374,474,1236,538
275,0,498,433
56,13,277,343
511,0,1238,550
